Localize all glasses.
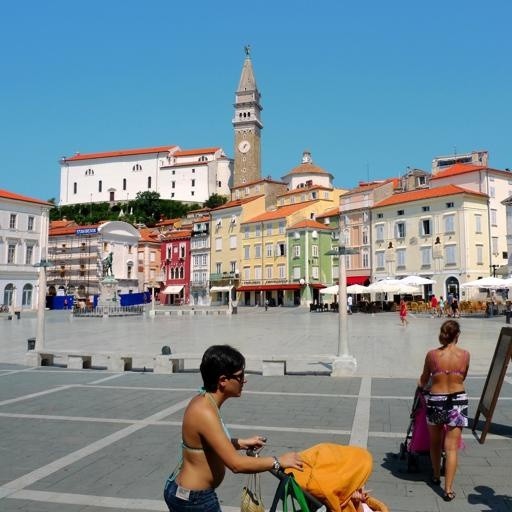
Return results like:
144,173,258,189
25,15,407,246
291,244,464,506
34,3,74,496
228,371,244,382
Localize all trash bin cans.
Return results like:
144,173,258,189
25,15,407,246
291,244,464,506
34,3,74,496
15,312,20,319
28,338,35,350
232,307,237,314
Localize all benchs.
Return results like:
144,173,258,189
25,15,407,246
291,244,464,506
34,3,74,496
152,352,202,373
27,349,133,372
0,313,12,320
259,355,357,377
149,309,231,316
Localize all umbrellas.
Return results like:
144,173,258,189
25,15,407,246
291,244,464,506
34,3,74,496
319,275,437,310
460,277,512,297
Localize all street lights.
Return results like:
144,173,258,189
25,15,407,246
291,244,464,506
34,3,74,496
31,246,55,351
324,234,364,375
229,271,235,284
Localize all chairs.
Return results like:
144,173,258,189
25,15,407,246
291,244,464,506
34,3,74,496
308,299,512,315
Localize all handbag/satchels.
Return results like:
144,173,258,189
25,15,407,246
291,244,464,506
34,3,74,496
241,487,265,512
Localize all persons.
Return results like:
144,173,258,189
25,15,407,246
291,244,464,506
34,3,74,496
417,320,470,501
430,292,460,318
63,299,92,314
164,345,303,512
398,300,409,326
491,292,496,305
351,484,381,512
348,294,353,314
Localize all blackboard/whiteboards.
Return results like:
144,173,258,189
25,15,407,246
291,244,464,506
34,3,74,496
479,327,512,418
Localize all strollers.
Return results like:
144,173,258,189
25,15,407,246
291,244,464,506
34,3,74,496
401,383,463,473
245,436,389,512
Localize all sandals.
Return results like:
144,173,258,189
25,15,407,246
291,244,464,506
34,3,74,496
432,477,440,487
444,492,455,501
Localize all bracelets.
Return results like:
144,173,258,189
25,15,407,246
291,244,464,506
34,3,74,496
272,456,281,470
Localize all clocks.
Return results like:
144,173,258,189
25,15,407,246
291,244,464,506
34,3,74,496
238,140,250,153
